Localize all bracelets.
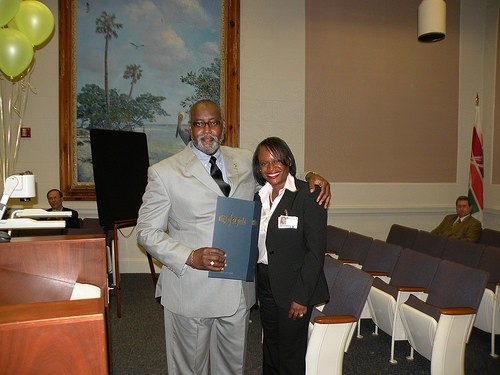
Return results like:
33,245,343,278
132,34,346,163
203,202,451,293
191,250,194,269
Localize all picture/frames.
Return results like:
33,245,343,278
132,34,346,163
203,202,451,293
59,0,240,201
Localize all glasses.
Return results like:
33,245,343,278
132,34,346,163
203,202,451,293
194,120,220,128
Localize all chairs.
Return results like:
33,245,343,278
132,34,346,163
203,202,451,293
305,224,500,375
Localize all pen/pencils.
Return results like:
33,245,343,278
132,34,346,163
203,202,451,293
285,209,288,216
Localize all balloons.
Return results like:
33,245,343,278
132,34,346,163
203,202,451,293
0,0,56,80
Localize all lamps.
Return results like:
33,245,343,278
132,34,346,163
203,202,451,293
418,0,446,43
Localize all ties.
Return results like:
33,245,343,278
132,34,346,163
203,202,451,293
209,156,231,197
456,217,461,223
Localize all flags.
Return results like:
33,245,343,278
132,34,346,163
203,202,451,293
468,105,484,223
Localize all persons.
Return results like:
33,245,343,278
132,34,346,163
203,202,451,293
429,196,484,241
40,189,81,228
280,217,286,225
137,99,332,375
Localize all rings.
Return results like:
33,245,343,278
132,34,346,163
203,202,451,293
210,261,214,266
300,313,304,317
